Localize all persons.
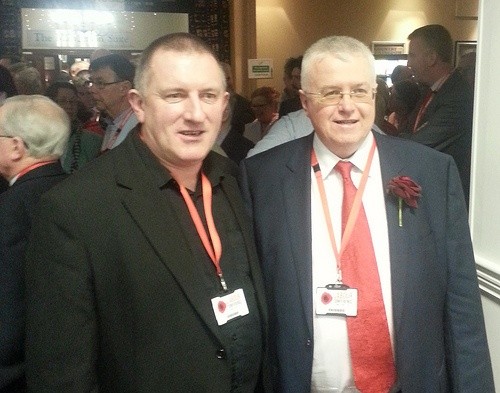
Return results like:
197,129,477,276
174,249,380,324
219,62,255,123
217,100,256,162
279,56,303,117
399,25,473,182
44,82,103,173
0,94,71,393
375,65,420,138
459,49,475,94
88,55,140,151
237,35,495,393
24,32,269,393
0,58,42,104
69,49,113,135
242,87,279,142
247,109,386,158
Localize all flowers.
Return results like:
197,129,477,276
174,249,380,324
386,176,421,228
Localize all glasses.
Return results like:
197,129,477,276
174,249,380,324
85,76,124,89
305,84,377,106
247,102,268,113
56,95,78,106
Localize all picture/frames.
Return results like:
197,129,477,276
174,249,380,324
454,40,477,68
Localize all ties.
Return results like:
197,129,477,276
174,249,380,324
334,161,397,393
414,87,434,134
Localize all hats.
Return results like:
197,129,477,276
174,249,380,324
70,61,90,77
